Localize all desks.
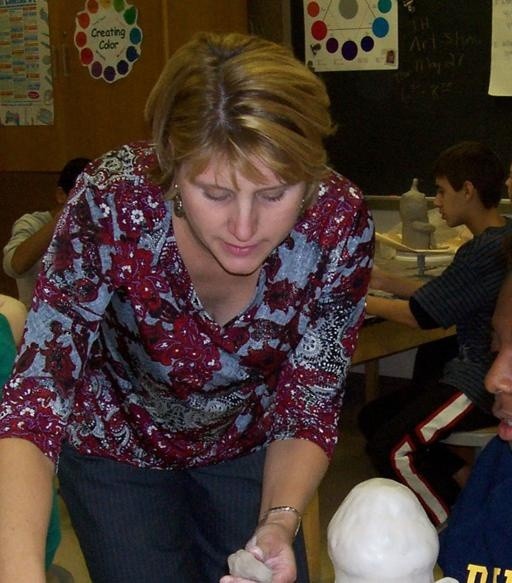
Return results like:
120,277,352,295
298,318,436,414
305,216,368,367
346,252,457,401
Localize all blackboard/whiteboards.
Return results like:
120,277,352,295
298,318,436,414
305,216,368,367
290,0,512,211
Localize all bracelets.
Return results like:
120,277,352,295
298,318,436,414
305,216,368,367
258,505,303,536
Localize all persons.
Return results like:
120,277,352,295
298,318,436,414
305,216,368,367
0,313,62,572
3,158,94,308
1,31,375,583
436,270,512,583
359,140,511,530
